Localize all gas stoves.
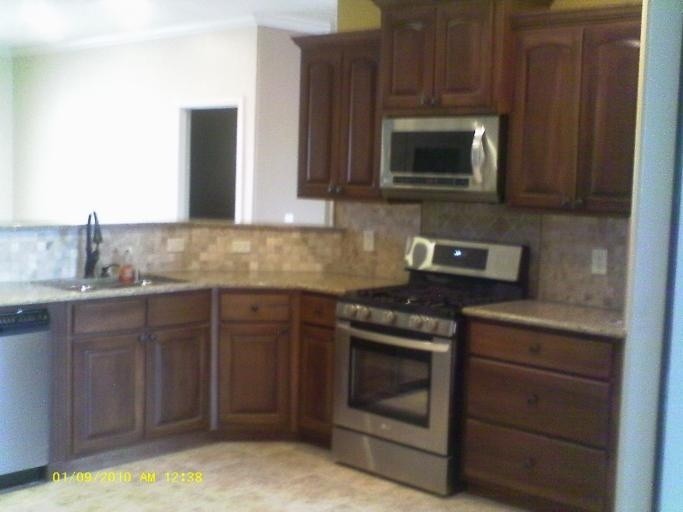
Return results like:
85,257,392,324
335,281,520,338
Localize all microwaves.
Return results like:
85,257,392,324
377,115,506,206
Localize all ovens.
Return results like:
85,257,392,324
329,323,468,498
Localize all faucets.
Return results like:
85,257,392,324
86,209,103,276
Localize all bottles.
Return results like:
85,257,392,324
119,247,137,285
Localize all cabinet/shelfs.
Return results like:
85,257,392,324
463,319,621,512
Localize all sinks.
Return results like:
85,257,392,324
31,274,191,293
